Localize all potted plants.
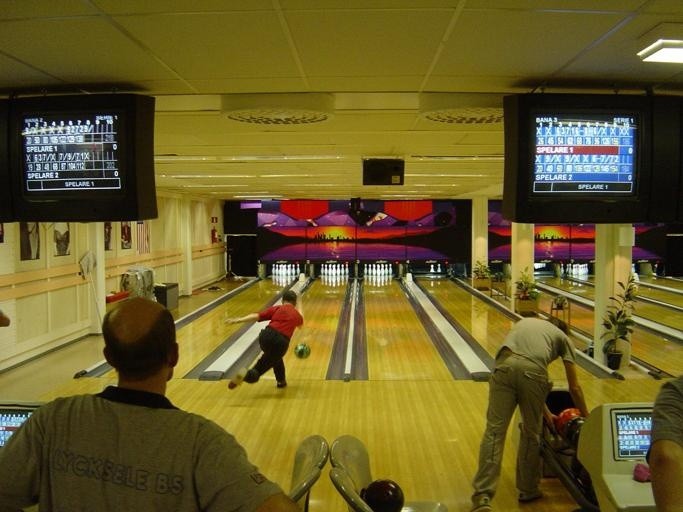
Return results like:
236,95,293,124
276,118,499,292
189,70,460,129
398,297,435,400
514,266,543,316
599,273,639,370
554,294,568,309
472,261,492,291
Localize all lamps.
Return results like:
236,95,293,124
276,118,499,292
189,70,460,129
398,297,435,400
635,22,683,65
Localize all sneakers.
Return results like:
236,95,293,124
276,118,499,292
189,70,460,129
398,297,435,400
471,497,491,512
519,489,543,503
228,367,246,389
278,380,286,387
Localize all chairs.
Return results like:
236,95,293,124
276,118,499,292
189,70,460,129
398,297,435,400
329,435,448,512
287,435,328,512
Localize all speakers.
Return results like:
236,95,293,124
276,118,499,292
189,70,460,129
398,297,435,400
363,158,404,186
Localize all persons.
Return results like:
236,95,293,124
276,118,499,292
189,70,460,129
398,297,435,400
471,317,589,512
225,290,303,389
0,296,301,512
645,375,683,512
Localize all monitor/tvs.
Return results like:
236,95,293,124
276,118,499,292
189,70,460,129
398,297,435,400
0,403,47,450
503,94,646,223
612,404,655,461
1,94,159,221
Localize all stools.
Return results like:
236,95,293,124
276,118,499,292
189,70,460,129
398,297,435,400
153,282,180,311
